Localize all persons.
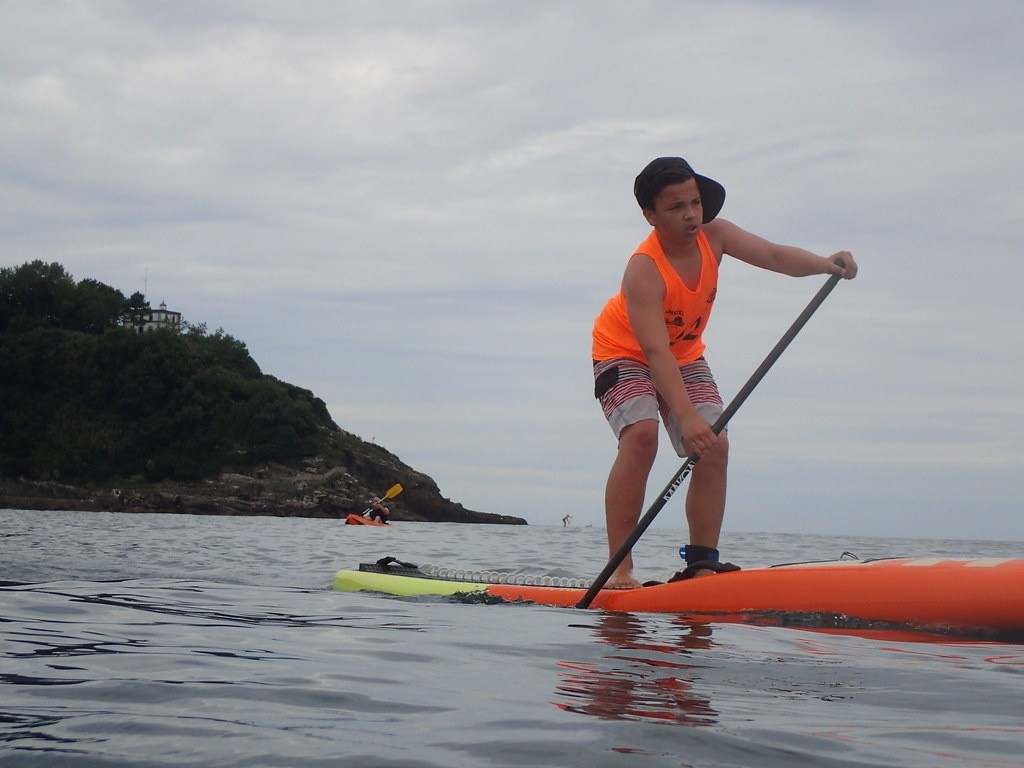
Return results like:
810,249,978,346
563,514,569,527
592,157,858,589
366,497,391,524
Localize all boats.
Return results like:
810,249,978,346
345,513,391,527
331,555,1023,647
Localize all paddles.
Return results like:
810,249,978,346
360,483,403,518
574,272,842,610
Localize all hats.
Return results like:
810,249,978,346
634,157,726,225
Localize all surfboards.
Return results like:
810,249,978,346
344,511,389,526
333,550,1024,648
